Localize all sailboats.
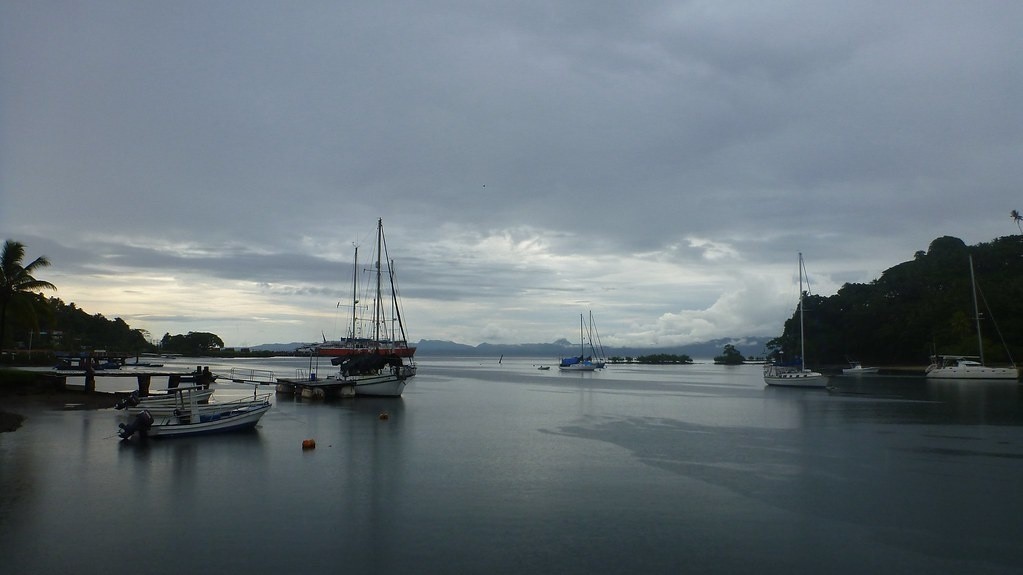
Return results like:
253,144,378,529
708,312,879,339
314,216,417,358
331,242,417,396
762,252,831,387
924,253,1023,379
559,310,607,371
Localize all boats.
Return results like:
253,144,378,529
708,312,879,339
117,383,272,439
114,384,215,415
925,337,977,373
841,355,880,374
538,365,550,370
